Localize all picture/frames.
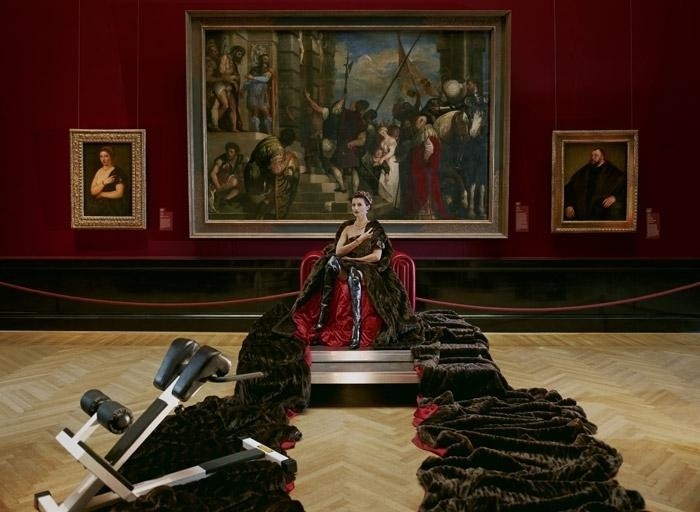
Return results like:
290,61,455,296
187,7,516,241
549,128,640,234
70,127,148,231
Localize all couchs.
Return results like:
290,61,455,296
299,247,416,348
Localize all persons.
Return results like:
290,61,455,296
291,190,413,348
82,144,133,217
561,147,627,221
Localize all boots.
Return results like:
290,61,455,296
348,274,361,349
315,256,340,329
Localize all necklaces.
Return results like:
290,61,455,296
353,221,369,230
103,166,112,171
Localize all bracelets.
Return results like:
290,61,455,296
354,239,360,248
102,180,106,186
